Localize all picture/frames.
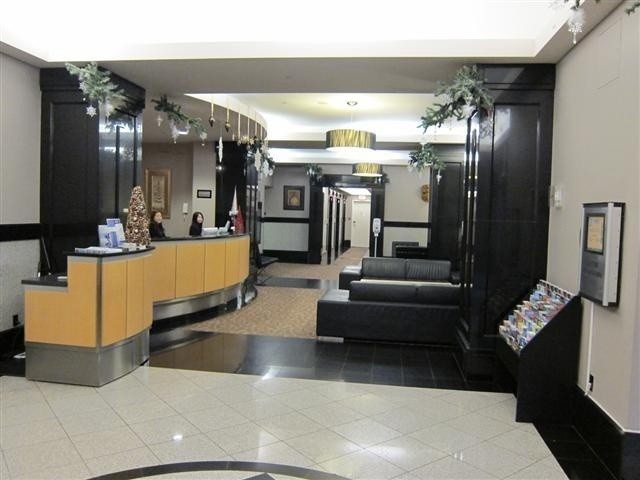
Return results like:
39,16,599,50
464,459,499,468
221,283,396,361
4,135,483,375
144,167,171,219
283,185,305,211
197,189,212,199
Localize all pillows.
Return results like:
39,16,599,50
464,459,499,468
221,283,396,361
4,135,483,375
348,280,417,304
416,286,458,306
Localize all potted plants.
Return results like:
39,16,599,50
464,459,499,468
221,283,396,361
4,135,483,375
254,150,281,178
417,63,496,135
303,163,323,186
407,144,448,177
370,171,389,195
105,110,134,135
65,62,127,118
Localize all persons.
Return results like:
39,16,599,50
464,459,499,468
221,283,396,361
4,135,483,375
189,212,204,235
148,209,166,237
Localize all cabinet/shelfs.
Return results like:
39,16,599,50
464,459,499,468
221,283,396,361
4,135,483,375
455,64,557,380
427,143,466,260
39,67,147,280
214,140,256,269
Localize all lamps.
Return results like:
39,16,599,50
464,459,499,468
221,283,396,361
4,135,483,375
547,0,603,45
352,163,384,178
325,101,377,153
154,93,208,147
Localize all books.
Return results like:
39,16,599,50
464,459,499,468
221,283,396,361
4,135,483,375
499,285,569,353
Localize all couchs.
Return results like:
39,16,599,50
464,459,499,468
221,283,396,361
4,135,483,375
391,240,428,258
314,257,462,347
